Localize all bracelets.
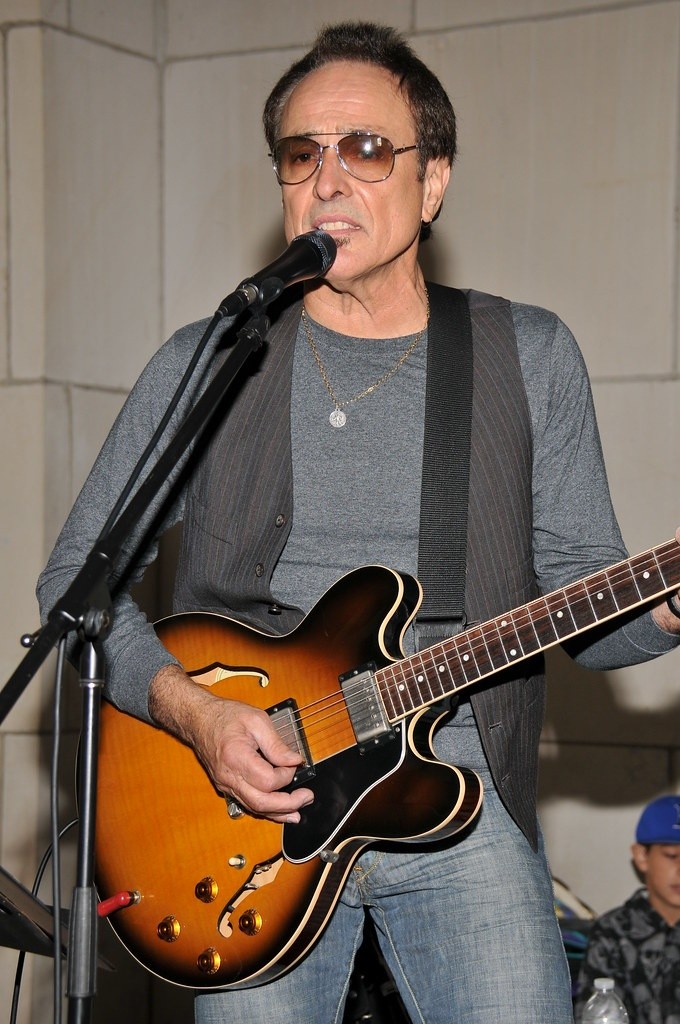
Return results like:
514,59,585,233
666,597,680,619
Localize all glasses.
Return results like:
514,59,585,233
267,132,430,184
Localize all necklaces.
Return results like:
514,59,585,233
301,287,430,427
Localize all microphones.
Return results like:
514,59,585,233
213,230,337,319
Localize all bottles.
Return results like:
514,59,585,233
580,977,630,1024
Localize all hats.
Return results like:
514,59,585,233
637,795,680,846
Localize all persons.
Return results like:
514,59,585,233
37,21,680,1024
575,795,680,1024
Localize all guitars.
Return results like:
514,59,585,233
71,539,680,992
